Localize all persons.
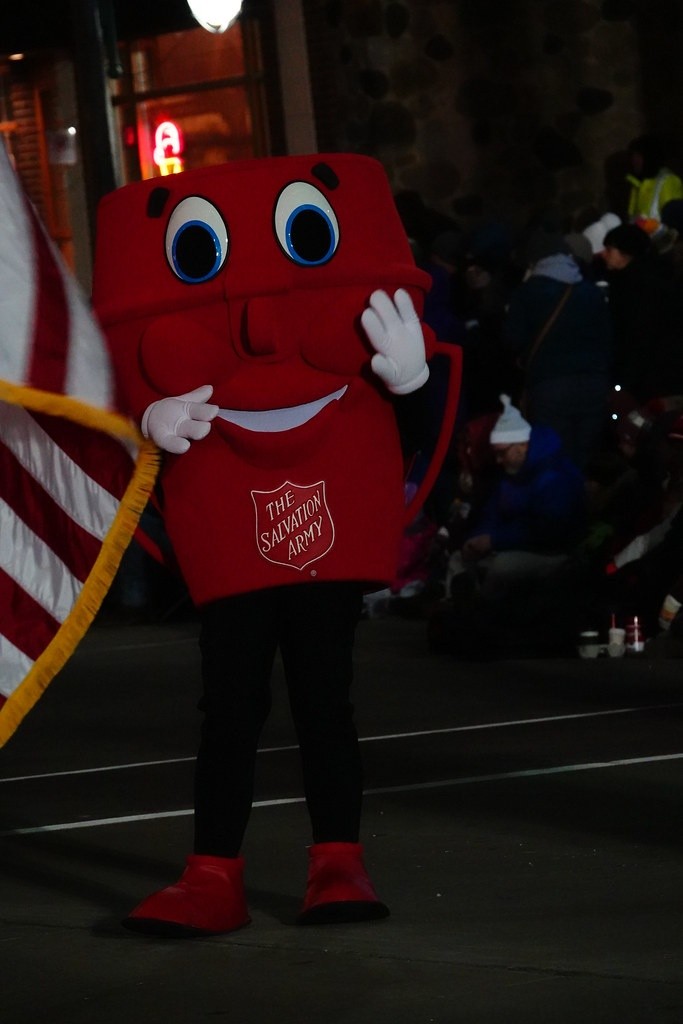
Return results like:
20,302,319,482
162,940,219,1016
394,135,683,606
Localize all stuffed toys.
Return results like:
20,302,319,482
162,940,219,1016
89,154,438,935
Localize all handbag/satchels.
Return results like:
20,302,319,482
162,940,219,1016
518,397,530,420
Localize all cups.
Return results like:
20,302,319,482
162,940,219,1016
607,627,625,658
625,624,646,653
581,630,604,659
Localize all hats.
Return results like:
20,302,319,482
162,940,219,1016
490,394,531,445
604,225,651,256
428,230,466,263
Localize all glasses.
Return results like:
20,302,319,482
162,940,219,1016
495,445,511,457
